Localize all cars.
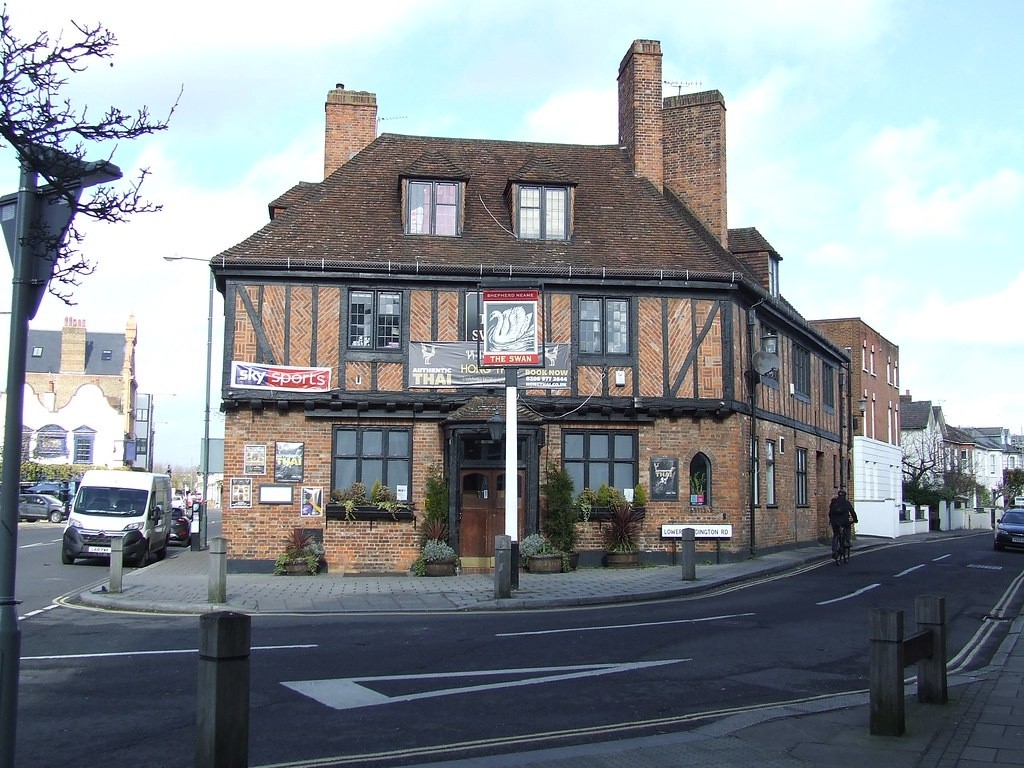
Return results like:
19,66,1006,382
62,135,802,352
994,509,1024,552
170,508,193,542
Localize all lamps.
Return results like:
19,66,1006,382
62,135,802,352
760,336,778,354
487,407,505,442
853,396,868,418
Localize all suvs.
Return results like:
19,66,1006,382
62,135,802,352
17,493,71,523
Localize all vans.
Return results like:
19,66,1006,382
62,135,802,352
61,468,172,568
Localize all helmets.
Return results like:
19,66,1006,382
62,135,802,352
838,491,847,496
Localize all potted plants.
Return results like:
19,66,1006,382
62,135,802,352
601,503,643,568
541,454,579,569
518,530,571,575
688,471,706,505
327,480,413,522
271,525,325,578
574,484,647,521
414,518,462,576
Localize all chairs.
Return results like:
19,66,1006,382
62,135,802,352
94,498,110,510
114,500,130,512
133,500,145,513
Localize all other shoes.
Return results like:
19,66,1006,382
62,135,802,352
832,551,835,557
846,543,850,548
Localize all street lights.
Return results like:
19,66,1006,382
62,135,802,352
0,140,126,768
163,256,213,550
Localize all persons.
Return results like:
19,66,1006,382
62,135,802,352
828,490,858,559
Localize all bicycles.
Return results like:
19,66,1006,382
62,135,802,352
832,520,858,566
